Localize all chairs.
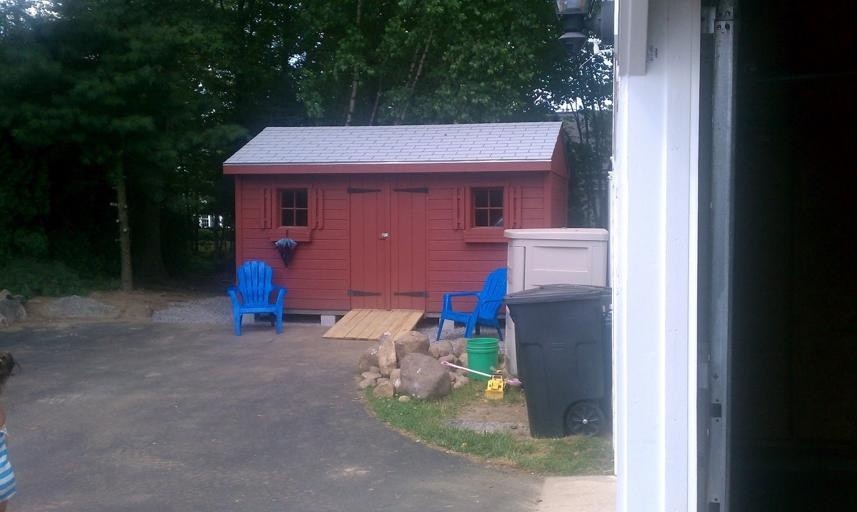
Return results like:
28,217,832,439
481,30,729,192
227,260,288,335
436,267,507,342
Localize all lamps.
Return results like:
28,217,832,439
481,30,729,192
556,0,613,54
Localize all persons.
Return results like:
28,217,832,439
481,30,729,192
1,403,17,509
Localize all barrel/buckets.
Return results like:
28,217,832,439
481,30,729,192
467,338,499,379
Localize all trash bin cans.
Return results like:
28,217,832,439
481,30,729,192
503,284,612,439
